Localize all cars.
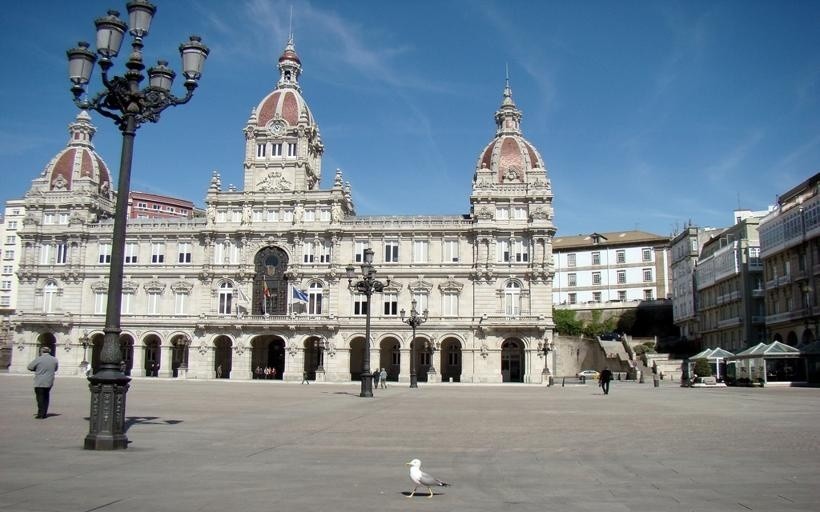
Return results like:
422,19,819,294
576,370,601,379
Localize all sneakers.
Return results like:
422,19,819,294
35,414,48,419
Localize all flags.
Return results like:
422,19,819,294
291,287,309,302
263,281,271,299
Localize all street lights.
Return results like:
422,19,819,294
79,330,92,366
66,0,210,450
400,299,429,388
537,337,554,375
345,248,391,397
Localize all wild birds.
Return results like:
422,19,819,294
404,458,450,499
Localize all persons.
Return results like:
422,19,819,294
598,366,613,395
27,346,58,420
373,367,389,389
255,365,277,380
217,364,223,378
301,369,310,385
